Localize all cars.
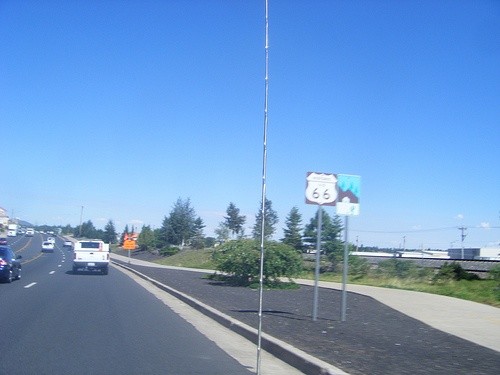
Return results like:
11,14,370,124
18,225,57,237
0,246,22,283
0,238,7,244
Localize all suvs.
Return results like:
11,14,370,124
42,240,54,252
73,239,110,274
63,240,72,248
26,229,34,236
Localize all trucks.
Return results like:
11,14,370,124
8,224,17,237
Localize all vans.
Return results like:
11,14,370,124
46,236,55,244
